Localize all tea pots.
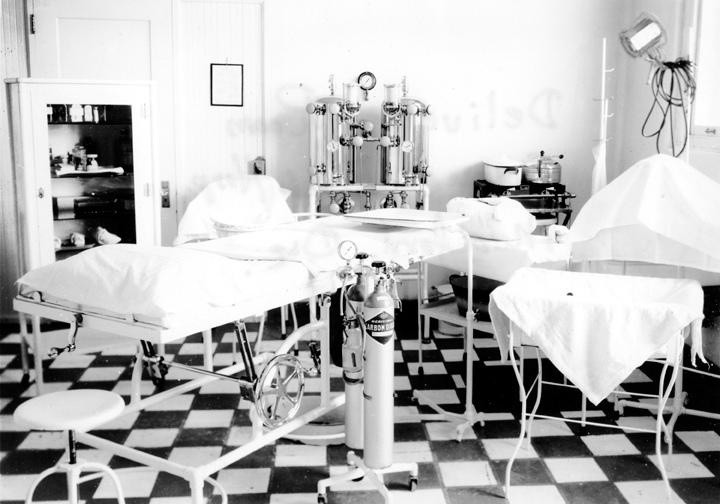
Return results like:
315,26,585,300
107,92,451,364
524,150,562,184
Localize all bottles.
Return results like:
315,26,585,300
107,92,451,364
72,146,98,171
83,105,100,123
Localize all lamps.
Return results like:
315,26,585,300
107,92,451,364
618,12,693,94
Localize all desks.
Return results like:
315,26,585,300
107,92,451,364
487,266,710,504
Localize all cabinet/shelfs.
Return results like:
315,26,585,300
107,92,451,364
6,71,162,276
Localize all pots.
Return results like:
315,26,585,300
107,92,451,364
482,160,523,188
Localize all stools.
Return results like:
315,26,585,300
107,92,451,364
17,383,127,504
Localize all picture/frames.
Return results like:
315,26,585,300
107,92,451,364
210,63,245,106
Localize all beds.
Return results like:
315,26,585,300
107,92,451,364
10,212,486,504
412,210,720,371
